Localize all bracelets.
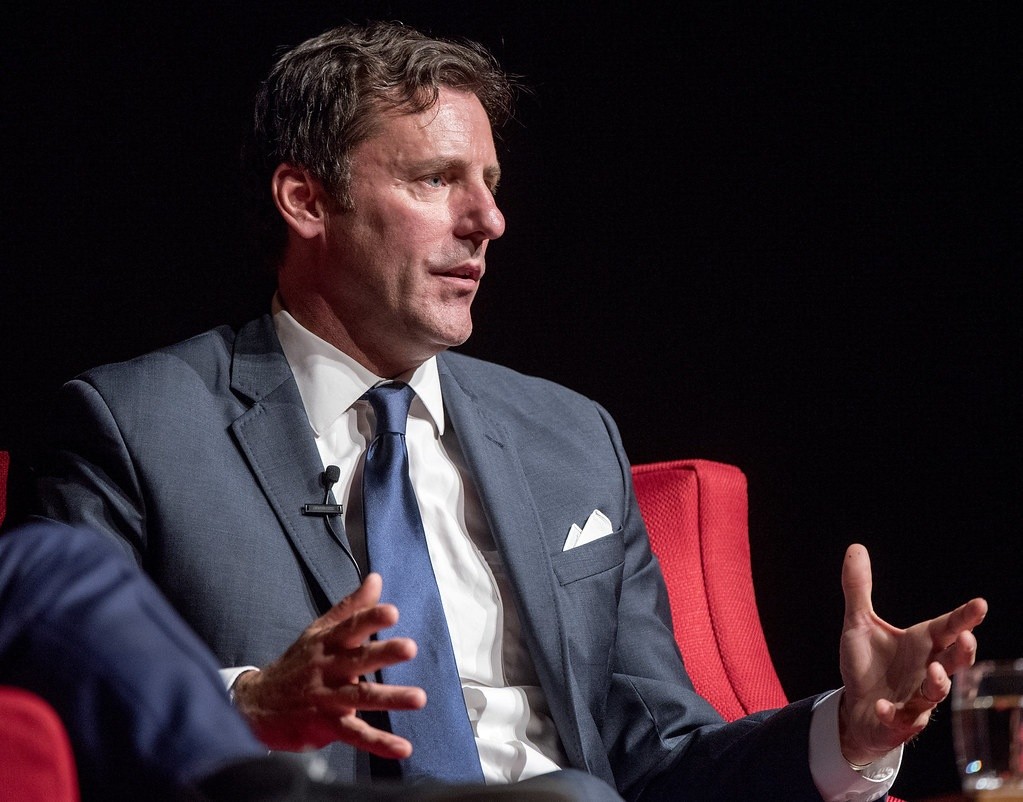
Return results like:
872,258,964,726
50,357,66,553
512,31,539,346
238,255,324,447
841,754,874,771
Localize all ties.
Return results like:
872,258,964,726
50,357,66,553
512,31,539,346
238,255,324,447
361,385,485,785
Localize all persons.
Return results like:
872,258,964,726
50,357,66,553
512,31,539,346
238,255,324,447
25,20,988,802
0,527,573,802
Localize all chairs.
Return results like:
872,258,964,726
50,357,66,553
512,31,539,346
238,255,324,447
1,451,915,802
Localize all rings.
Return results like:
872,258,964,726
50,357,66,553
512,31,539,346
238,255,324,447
920,677,951,705
